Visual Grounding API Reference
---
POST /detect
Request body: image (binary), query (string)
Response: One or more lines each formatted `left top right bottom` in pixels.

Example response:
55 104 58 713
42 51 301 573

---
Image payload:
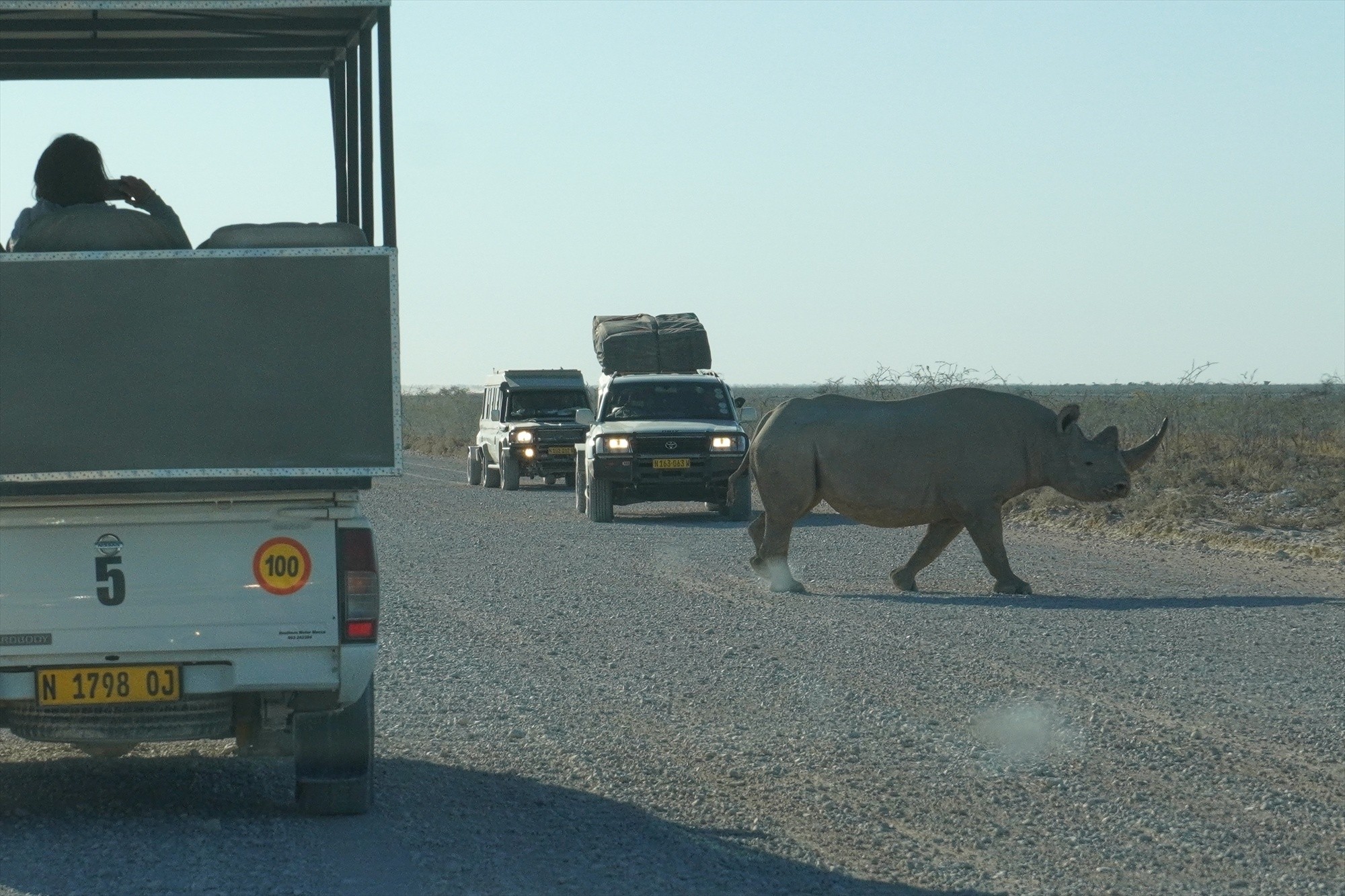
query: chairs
13 203 190 254
206 220 368 250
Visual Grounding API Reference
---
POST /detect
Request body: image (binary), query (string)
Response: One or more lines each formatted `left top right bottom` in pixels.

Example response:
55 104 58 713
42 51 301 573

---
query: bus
0 1 400 817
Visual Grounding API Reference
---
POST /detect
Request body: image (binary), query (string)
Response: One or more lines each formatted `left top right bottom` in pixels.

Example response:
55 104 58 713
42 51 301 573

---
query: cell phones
105 180 131 201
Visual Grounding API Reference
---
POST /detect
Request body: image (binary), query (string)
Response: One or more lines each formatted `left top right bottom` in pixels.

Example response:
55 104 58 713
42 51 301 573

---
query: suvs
573 369 753 523
467 368 595 491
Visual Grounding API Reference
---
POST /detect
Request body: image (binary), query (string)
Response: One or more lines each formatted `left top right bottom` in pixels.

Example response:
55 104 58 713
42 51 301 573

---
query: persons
5 133 192 252
612 388 656 417
